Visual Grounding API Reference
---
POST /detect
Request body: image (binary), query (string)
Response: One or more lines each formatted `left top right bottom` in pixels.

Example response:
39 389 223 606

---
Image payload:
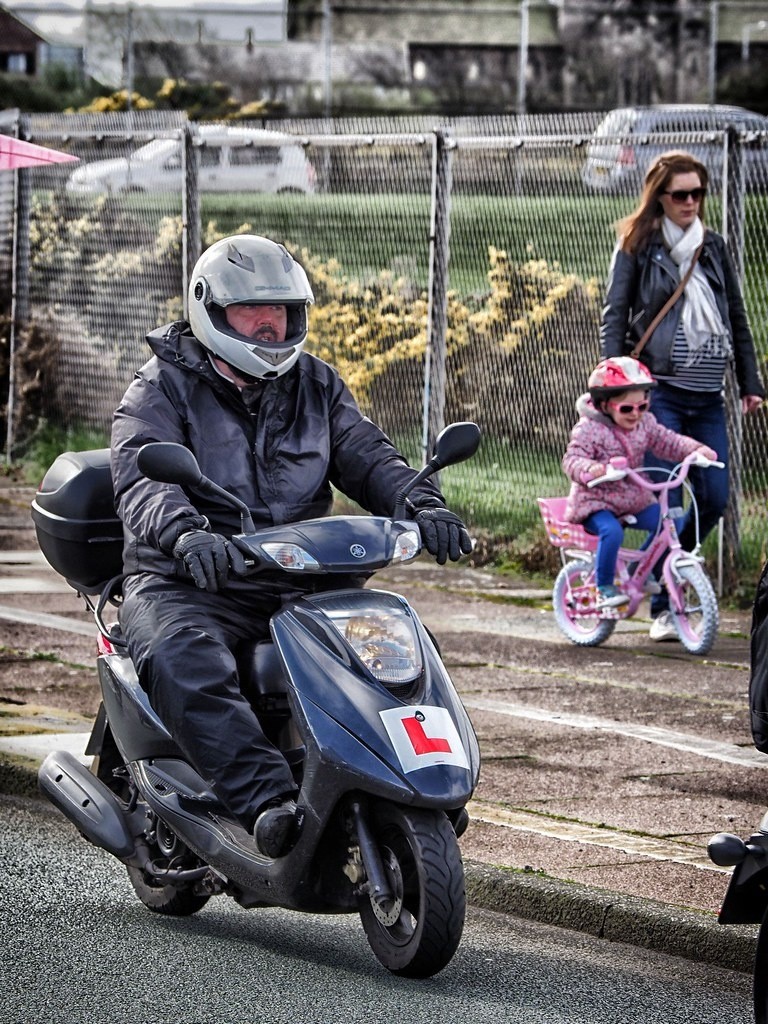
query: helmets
588 356 658 390
188 235 315 379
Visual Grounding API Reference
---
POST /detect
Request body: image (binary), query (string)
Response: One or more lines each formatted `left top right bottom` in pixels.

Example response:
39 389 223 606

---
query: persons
561 356 723 612
106 234 477 862
601 149 768 643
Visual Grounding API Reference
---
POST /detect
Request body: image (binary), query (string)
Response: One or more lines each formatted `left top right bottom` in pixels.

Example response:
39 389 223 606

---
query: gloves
172 528 247 591
413 508 472 566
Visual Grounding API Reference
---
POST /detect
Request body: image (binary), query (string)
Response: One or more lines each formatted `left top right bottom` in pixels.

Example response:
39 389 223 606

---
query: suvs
579 100 768 195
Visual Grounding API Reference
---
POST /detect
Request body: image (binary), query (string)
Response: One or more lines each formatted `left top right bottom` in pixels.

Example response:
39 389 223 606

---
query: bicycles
536 450 721 657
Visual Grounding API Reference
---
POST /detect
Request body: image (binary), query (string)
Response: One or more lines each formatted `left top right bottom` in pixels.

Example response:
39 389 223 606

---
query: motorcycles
32 422 483 979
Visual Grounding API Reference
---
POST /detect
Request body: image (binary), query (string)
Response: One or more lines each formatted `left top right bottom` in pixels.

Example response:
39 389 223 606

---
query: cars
65 125 316 215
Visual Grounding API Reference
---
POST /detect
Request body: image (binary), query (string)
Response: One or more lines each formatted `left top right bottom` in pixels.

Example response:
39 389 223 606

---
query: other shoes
452 807 469 838
254 795 302 859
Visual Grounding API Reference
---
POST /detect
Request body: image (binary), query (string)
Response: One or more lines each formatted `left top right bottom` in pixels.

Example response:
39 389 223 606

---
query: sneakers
627 562 661 593
650 609 681 641
595 584 630 611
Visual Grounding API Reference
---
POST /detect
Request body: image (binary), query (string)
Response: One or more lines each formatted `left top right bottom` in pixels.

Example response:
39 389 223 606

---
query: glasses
607 400 649 414
664 187 707 203
